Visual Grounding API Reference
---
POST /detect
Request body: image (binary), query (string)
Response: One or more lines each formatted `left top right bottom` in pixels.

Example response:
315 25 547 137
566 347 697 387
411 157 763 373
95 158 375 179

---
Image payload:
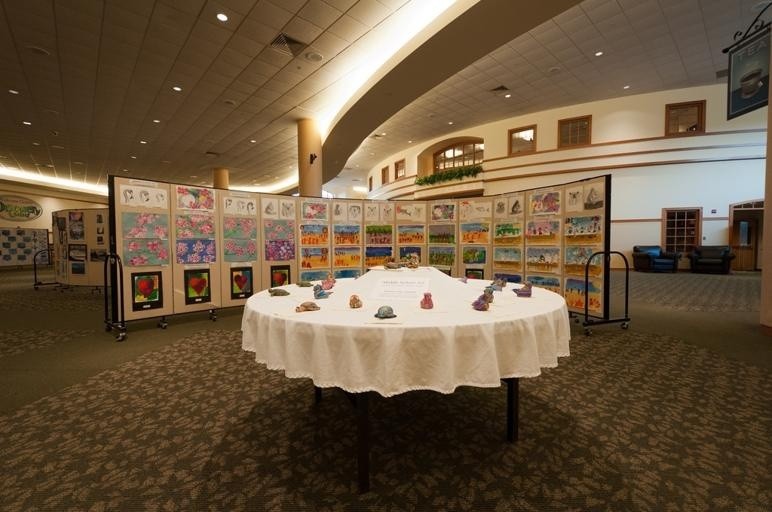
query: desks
240 266 572 443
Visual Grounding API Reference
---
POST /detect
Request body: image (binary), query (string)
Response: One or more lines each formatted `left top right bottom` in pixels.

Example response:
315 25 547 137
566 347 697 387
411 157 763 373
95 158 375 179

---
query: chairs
632 245 682 273
687 246 736 274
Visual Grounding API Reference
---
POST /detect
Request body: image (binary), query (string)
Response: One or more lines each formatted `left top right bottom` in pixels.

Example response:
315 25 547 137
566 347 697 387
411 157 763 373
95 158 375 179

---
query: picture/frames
726 27 771 123
